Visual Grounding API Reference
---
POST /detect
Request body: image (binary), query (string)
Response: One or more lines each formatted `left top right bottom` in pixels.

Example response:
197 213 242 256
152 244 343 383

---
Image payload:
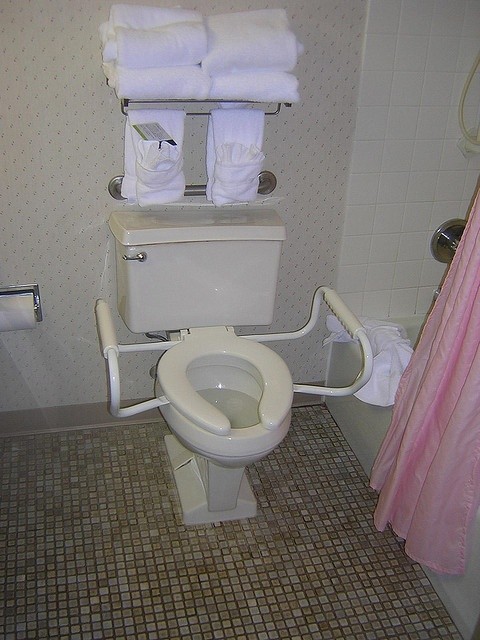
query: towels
199 62 302 109
96 3 206 40
100 58 209 102
321 309 424 408
201 5 299 45
204 107 268 208
119 107 189 208
198 33 303 73
96 22 206 75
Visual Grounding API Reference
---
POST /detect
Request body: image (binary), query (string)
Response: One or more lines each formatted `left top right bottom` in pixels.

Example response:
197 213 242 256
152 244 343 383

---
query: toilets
106 208 293 527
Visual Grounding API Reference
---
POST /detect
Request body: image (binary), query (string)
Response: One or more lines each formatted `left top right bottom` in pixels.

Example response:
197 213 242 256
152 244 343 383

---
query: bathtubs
324 313 480 638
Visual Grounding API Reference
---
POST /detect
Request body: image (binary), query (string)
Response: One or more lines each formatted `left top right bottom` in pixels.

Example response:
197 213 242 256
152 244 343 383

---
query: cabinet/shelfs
105 77 293 115
